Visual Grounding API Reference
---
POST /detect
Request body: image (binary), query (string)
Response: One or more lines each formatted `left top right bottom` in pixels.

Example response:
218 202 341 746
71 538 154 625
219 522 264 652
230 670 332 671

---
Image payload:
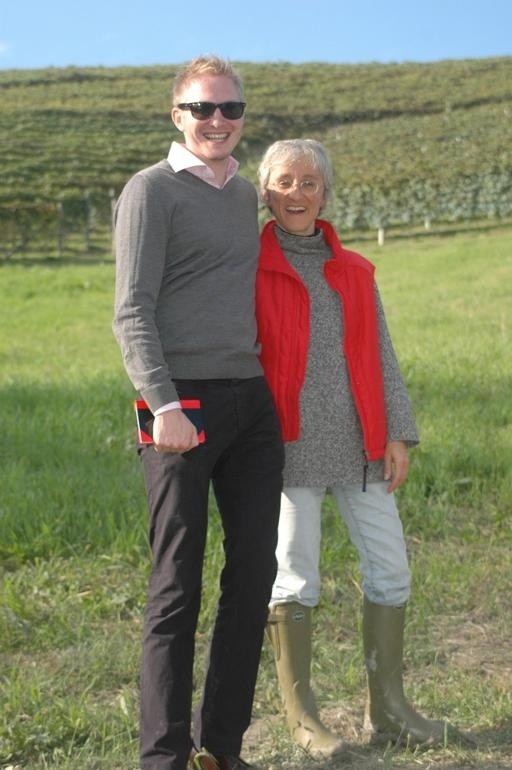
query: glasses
268 179 324 196
177 101 247 121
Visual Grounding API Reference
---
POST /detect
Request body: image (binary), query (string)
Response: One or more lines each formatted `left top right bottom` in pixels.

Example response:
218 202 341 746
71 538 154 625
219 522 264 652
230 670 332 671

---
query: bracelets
149 401 184 416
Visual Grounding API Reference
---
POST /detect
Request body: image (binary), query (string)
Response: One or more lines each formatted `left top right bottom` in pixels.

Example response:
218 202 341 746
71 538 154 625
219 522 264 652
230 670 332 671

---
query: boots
268 600 348 762
362 592 453 746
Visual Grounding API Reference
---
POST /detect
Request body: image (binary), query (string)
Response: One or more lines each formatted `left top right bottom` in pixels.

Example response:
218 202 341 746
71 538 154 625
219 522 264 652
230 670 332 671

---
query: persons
106 53 288 770
255 135 453 757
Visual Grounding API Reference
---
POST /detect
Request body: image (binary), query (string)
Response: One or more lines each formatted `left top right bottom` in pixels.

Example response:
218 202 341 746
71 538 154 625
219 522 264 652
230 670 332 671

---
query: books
133 399 206 446
